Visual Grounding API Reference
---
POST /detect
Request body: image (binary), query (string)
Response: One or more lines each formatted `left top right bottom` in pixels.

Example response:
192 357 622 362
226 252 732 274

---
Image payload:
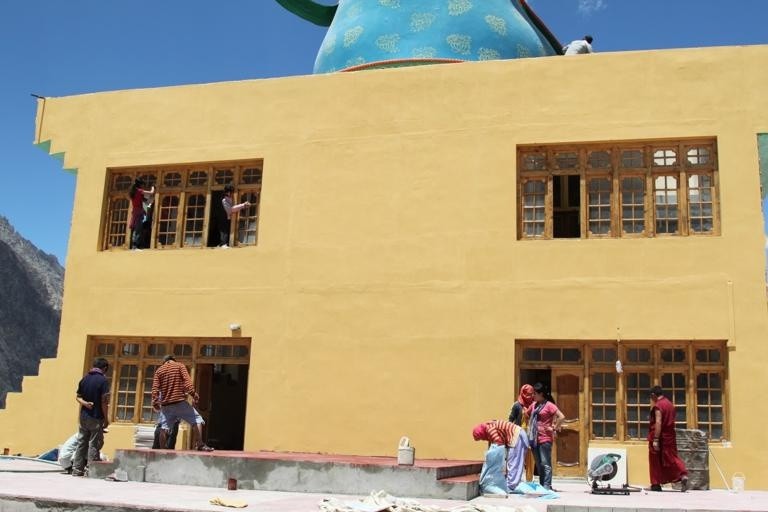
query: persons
129 177 155 250
71 358 110 476
508 384 536 482
58 429 109 474
473 420 530 491
152 354 214 452
525 382 565 489
562 35 593 54
643 386 689 492
220 184 251 246
152 411 178 448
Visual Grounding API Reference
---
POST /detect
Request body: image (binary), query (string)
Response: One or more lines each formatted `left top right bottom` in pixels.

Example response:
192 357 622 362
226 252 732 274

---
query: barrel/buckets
731 470 745 492
397 435 416 467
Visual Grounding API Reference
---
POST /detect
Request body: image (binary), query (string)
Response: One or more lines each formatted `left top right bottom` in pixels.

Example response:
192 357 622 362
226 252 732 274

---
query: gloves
153 404 161 412
192 393 200 406
652 439 660 452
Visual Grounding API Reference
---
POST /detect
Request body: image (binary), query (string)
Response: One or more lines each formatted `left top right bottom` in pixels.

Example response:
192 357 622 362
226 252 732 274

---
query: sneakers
71 471 84 476
643 485 662 491
196 443 214 451
681 474 689 492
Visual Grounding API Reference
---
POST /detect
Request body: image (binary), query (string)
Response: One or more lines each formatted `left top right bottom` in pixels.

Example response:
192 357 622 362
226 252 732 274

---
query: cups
4 448 9 455
228 479 237 490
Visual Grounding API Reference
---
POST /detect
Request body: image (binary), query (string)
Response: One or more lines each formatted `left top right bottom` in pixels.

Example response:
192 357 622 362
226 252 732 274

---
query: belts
163 400 185 406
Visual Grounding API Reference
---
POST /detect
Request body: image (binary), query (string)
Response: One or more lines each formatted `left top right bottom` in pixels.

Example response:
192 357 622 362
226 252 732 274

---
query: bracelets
552 426 555 431
653 437 659 439
654 439 660 442
